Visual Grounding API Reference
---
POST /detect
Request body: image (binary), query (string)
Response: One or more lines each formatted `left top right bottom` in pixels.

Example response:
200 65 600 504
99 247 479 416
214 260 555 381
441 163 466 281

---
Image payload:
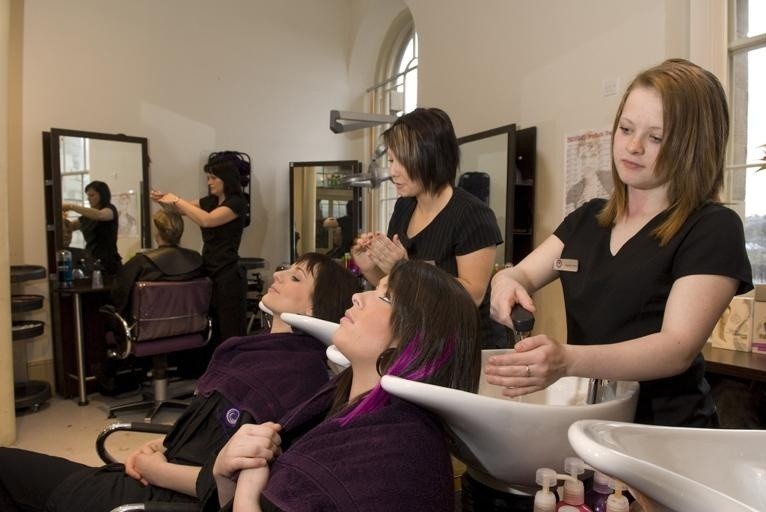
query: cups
56 249 73 288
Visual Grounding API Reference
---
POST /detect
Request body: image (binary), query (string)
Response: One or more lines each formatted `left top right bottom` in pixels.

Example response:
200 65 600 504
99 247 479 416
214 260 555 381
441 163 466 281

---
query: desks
47 273 104 408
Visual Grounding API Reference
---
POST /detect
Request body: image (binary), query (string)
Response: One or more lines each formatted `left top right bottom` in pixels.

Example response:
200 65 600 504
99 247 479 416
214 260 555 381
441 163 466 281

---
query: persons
19 254 355 512
61 220 85 269
150 155 259 349
566 135 618 217
492 56 753 427
349 107 503 309
59 181 124 272
322 197 355 256
107 204 203 341
210 258 479 508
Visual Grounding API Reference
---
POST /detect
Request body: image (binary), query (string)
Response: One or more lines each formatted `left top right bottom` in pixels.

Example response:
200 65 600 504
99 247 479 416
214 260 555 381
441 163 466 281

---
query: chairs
98 276 213 423
95 423 205 512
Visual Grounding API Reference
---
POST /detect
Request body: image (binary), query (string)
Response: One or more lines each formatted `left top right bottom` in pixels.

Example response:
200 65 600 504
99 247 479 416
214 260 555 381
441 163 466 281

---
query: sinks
326 345 639 485
568 420 766 512
259 302 338 347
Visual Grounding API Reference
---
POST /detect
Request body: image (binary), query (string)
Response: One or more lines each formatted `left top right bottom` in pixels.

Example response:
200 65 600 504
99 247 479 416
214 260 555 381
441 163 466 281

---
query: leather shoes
95 365 115 393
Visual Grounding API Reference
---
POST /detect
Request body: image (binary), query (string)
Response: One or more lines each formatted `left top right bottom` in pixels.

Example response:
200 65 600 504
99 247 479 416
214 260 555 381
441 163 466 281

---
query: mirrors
42 127 152 278
288 160 362 266
451 123 515 270
